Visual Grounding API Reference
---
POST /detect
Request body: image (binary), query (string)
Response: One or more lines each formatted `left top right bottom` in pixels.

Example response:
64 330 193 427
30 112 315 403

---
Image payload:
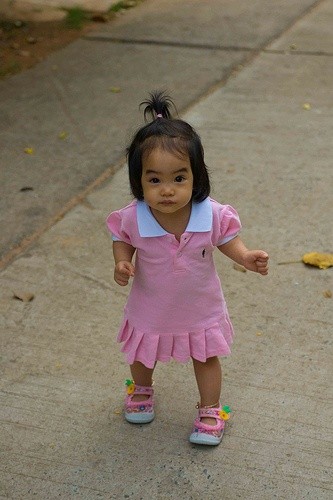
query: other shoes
123 380 155 424
189 402 232 445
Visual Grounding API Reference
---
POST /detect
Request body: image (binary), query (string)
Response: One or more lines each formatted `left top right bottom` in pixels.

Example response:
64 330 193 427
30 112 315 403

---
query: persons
104 87 271 445
